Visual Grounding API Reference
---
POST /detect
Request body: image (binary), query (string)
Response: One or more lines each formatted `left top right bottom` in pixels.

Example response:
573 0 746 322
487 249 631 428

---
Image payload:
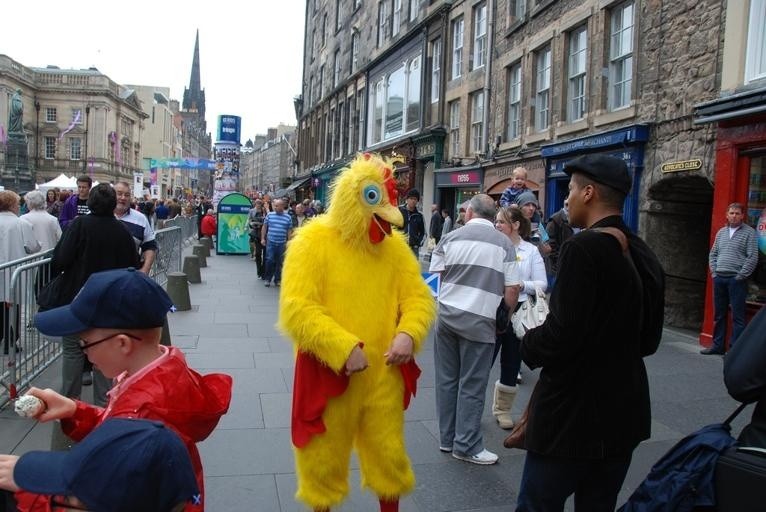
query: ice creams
15 395 47 417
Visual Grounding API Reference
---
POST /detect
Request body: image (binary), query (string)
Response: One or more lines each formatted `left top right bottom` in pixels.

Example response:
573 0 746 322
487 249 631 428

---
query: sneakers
440 446 499 464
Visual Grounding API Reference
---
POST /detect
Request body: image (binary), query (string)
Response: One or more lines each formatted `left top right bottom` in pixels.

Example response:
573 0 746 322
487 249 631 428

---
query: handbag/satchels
511 281 550 340
617 424 737 512
37 270 70 308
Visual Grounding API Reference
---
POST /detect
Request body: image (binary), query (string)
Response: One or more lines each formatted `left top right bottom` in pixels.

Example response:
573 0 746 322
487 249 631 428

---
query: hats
35 267 173 336
13 418 199 512
563 155 632 195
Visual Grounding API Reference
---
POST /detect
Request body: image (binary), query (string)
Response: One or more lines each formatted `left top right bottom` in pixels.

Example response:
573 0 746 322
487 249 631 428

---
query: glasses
78 333 141 354
50 495 87 512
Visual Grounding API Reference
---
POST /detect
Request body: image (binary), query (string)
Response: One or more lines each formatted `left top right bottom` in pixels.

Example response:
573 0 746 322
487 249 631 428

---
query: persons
1 268 234 511
51 181 160 456
129 193 196 230
430 204 467 259
700 204 759 355
500 167 581 294
247 188 323 288
279 157 435 511
196 192 217 247
6 86 26 133
491 206 548 428
723 305 766 512
430 194 521 467
399 188 427 260
0 176 93 354
521 155 667 512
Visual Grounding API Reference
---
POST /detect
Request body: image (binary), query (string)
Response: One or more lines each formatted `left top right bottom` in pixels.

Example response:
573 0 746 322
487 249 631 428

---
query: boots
492 380 518 429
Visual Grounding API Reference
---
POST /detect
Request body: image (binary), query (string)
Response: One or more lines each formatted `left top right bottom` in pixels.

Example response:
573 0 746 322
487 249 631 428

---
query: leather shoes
700 346 725 354
